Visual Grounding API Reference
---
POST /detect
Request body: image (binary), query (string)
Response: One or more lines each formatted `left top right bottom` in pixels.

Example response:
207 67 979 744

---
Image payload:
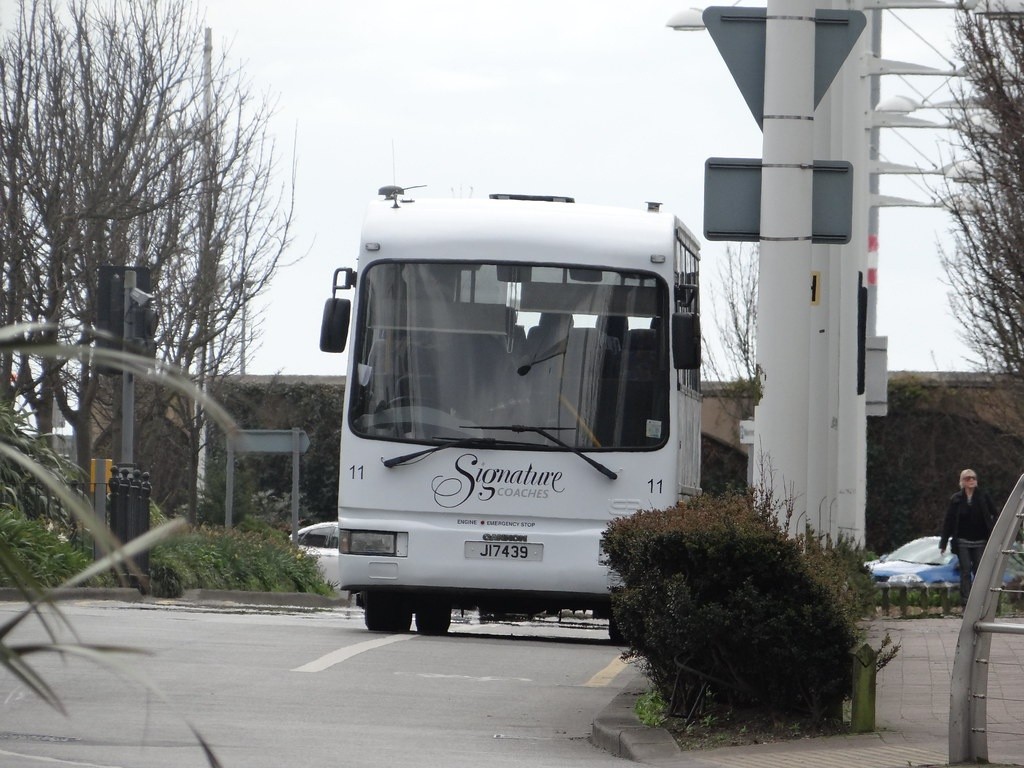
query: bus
319 179 701 651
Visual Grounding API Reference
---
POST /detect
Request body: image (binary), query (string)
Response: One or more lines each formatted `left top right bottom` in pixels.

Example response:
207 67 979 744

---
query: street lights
664 0 1023 568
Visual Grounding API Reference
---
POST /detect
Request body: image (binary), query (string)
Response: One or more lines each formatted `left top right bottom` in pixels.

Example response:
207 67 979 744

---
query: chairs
494 310 661 381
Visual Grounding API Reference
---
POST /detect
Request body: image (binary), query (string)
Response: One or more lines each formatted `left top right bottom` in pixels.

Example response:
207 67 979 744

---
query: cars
288 520 349 600
862 534 1021 590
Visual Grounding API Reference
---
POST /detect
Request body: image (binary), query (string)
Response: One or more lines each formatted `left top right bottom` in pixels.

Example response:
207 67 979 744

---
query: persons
940 469 999 620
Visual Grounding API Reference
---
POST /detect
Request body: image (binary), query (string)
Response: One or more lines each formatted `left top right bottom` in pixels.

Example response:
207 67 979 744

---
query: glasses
963 476 976 481
